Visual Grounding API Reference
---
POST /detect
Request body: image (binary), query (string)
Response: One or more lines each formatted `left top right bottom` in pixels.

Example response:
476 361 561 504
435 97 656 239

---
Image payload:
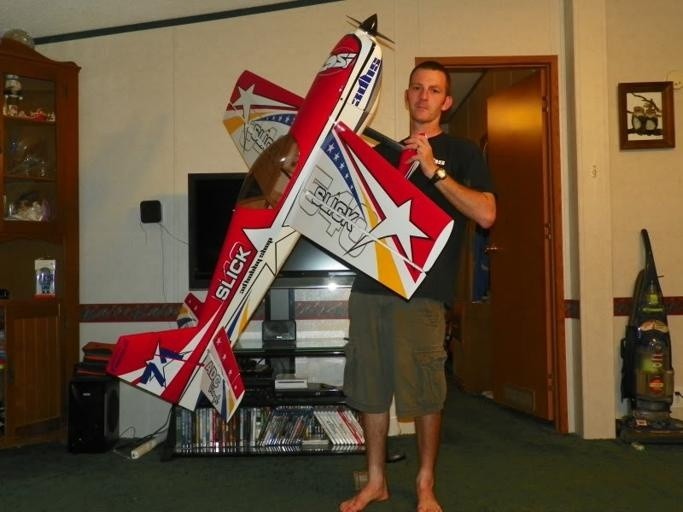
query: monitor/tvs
188 173 357 291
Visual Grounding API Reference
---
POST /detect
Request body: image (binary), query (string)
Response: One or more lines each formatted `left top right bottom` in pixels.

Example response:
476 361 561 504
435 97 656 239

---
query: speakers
67 375 119 454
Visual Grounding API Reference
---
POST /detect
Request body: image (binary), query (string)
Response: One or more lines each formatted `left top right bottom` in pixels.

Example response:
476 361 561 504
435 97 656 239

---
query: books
173 406 364 452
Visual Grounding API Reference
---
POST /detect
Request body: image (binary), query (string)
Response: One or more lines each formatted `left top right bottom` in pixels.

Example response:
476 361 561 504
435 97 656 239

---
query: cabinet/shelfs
0 36 79 450
169 338 368 461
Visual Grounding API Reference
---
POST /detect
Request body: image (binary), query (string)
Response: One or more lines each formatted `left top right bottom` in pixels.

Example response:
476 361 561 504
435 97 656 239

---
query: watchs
426 167 447 185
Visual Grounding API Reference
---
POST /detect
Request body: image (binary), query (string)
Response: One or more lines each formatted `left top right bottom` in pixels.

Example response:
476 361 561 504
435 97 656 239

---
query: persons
336 61 497 512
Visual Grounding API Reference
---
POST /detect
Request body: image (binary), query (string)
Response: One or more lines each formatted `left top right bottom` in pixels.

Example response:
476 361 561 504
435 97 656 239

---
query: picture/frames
617 80 676 150
